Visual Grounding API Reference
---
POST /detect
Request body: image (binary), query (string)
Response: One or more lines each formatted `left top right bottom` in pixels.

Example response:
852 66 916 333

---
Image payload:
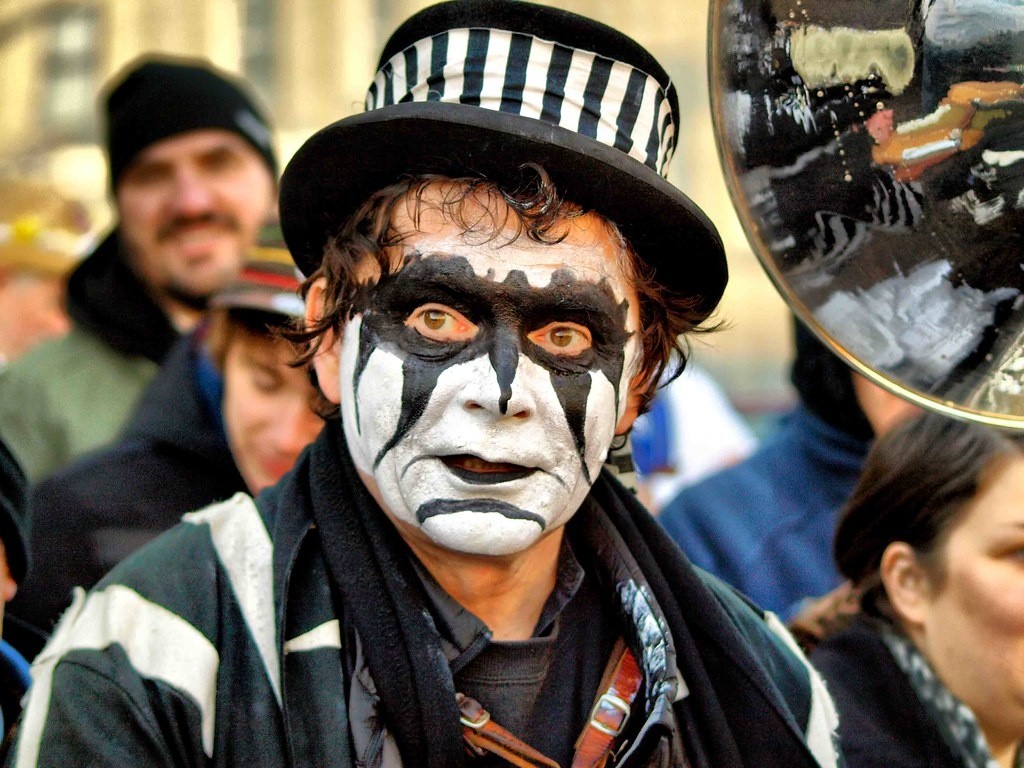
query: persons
0 1 919 768
647 306 918 628
782 403 1024 767
0 439 41 768
0 50 280 500
619 358 757 517
23 181 331 648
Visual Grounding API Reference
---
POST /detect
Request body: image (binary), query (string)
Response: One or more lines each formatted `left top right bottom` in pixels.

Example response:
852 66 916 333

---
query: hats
211 212 309 318
277 0 729 332
92 49 282 186
0 439 35 588
